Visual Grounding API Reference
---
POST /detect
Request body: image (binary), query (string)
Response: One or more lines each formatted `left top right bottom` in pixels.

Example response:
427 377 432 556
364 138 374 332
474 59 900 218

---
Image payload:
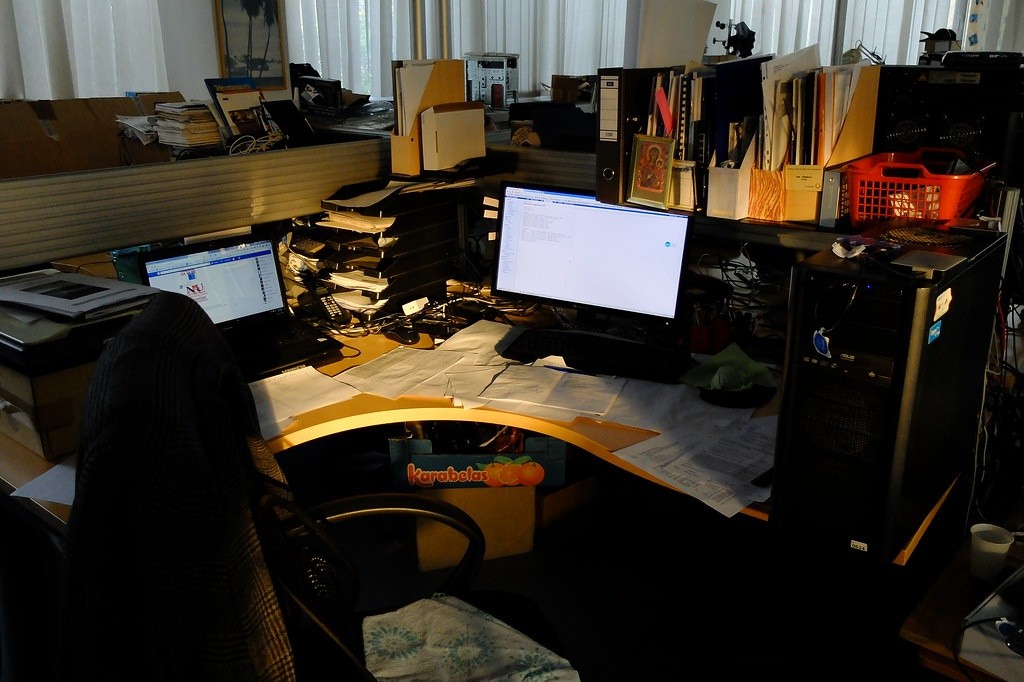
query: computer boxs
870 49 1024 194
467 55 519 111
768 217 1006 558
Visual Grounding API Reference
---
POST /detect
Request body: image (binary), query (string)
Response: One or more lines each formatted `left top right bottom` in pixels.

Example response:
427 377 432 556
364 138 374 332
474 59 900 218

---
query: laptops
138 233 344 384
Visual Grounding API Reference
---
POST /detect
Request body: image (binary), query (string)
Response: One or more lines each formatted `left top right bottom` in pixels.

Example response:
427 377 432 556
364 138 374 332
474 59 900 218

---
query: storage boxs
839 148 998 230
383 433 567 576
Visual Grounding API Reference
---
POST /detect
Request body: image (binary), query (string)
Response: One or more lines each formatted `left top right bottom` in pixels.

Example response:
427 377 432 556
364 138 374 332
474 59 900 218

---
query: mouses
385 326 420 345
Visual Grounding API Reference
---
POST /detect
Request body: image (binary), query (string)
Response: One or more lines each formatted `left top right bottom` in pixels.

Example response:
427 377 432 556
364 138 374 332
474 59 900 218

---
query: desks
309 116 393 137
0 281 964 566
899 538 1024 682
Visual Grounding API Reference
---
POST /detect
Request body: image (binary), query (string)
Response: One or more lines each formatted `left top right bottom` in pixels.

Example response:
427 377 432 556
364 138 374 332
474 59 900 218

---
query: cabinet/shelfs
325 181 489 323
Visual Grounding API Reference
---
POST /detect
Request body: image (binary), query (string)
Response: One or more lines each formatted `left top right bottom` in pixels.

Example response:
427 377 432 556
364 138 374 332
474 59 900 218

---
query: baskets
841 148 997 228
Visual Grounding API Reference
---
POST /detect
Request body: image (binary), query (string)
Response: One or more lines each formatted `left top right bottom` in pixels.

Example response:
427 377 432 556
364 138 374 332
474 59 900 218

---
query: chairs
67 291 580 682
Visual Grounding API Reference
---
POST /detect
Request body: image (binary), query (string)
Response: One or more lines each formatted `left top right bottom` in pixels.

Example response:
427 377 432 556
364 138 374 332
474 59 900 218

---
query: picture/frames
212 0 288 94
625 134 677 210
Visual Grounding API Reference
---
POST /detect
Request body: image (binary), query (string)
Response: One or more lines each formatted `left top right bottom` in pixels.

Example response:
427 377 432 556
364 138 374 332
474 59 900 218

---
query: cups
969 523 1015 583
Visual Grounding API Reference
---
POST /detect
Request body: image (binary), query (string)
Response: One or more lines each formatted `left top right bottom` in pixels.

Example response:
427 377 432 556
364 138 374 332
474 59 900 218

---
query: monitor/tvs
492 180 697 330
